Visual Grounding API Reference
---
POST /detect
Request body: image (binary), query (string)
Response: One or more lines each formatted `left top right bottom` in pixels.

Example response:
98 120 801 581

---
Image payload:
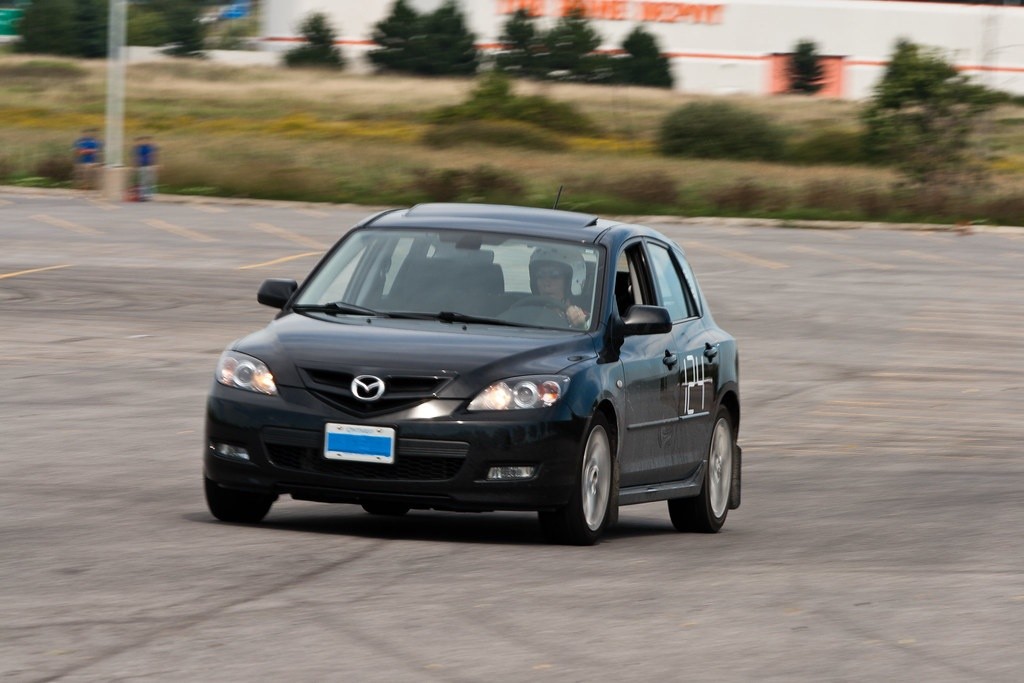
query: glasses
534 270 561 280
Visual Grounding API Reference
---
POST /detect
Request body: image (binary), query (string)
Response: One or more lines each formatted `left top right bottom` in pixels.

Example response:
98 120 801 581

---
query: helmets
529 244 586 297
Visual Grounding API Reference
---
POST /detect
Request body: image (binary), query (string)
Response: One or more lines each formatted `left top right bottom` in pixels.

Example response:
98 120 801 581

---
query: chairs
398 256 486 316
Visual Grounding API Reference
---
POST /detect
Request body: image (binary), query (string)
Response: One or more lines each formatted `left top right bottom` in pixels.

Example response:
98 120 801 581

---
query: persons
529 246 587 326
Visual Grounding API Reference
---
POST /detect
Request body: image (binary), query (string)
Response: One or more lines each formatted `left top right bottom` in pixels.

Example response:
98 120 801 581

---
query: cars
201 197 745 546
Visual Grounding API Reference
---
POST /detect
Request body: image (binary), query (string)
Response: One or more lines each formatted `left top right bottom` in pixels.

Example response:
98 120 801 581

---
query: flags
199 3 249 24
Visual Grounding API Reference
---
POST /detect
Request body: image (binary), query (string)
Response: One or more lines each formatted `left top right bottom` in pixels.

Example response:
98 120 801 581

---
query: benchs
470 263 633 321
